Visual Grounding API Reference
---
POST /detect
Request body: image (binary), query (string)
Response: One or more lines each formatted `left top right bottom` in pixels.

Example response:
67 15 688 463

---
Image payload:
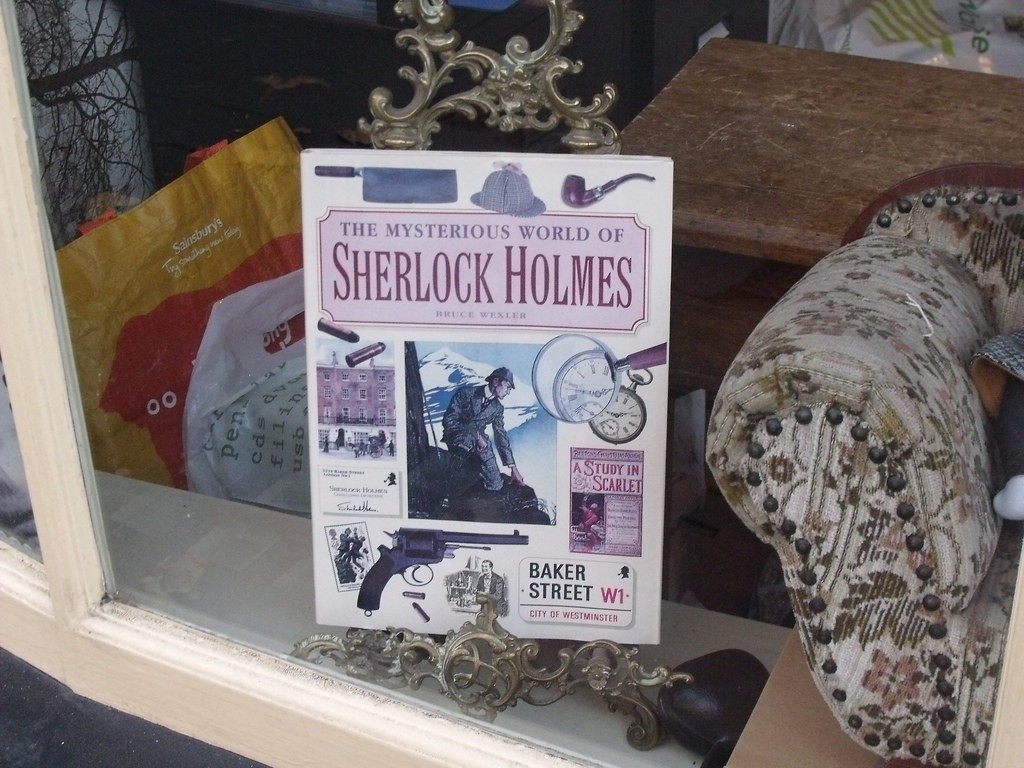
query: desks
618 34 1023 425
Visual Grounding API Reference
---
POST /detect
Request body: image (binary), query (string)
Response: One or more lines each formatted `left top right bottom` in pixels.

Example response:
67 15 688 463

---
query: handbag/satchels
778 0 1023 80
53 116 313 520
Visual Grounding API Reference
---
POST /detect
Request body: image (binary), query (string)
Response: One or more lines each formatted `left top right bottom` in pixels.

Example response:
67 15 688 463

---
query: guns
354 529 529 618
